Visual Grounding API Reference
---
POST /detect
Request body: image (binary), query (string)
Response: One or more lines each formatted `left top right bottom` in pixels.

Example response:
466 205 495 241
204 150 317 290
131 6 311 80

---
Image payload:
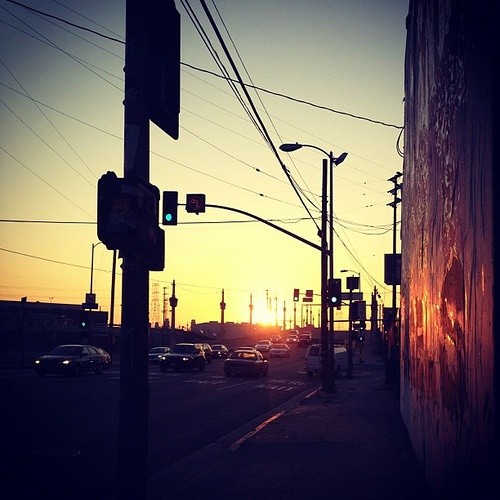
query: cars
269 334 283 343
269 343 290 357
148 346 170 365
34 344 106 376
224 347 268 379
286 329 314 348
255 340 273 352
210 344 228 358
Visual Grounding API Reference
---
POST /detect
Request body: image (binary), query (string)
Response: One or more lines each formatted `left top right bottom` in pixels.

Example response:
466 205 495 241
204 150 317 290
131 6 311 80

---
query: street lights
280 142 347 392
341 269 363 359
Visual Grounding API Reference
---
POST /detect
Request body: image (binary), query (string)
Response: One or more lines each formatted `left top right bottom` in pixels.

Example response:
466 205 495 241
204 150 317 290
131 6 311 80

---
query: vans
304 344 348 377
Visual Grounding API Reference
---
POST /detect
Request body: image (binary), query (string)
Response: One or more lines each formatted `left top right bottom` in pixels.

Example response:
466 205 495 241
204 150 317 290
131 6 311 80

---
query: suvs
159 343 205 372
193 342 213 363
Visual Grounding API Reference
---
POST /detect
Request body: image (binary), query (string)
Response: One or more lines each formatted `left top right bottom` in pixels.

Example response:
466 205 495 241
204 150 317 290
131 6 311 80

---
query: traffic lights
163 191 178 225
327 279 341 307
187 194 205 213
